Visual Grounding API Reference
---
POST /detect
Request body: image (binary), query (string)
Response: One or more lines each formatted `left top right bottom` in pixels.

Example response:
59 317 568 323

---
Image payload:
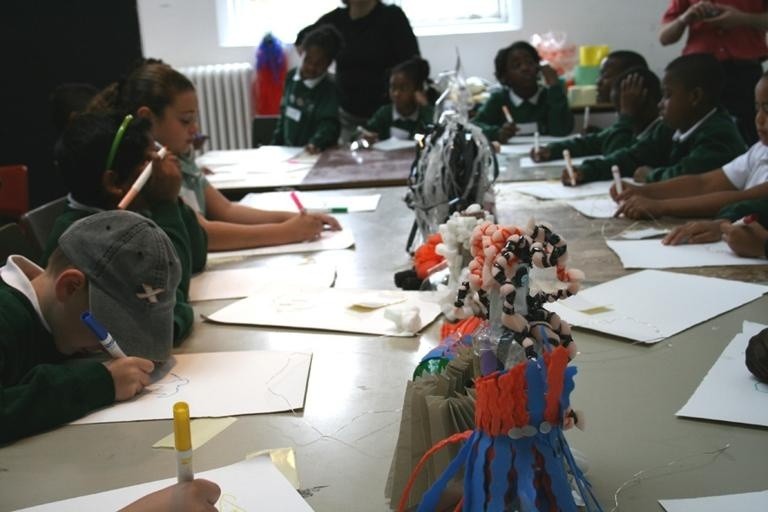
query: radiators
178 63 254 155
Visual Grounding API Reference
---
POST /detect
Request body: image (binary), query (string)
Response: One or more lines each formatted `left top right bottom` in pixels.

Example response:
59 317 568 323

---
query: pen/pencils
501 105 514 123
290 192 347 214
356 126 372 136
731 215 755 226
582 106 590 132
289 160 314 164
563 149 576 187
612 165 624 206
173 401 193 483
534 131 541 163
81 312 125 360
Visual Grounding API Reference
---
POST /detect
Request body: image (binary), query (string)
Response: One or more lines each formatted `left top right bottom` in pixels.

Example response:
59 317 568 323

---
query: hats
59 210 182 362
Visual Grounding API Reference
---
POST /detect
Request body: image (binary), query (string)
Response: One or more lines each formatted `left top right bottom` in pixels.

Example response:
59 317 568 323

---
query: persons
349 57 436 154
593 49 649 110
658 0 768 63
265 23 347 153
611 75 767 220
1 210 195 450
662 196 766 262
45 108 209 351
113 478 222 512
292 1 423 148
744 328 767 387
529 66 663 171
466 41 570 143
75 56 342 251
562 52 747 185
